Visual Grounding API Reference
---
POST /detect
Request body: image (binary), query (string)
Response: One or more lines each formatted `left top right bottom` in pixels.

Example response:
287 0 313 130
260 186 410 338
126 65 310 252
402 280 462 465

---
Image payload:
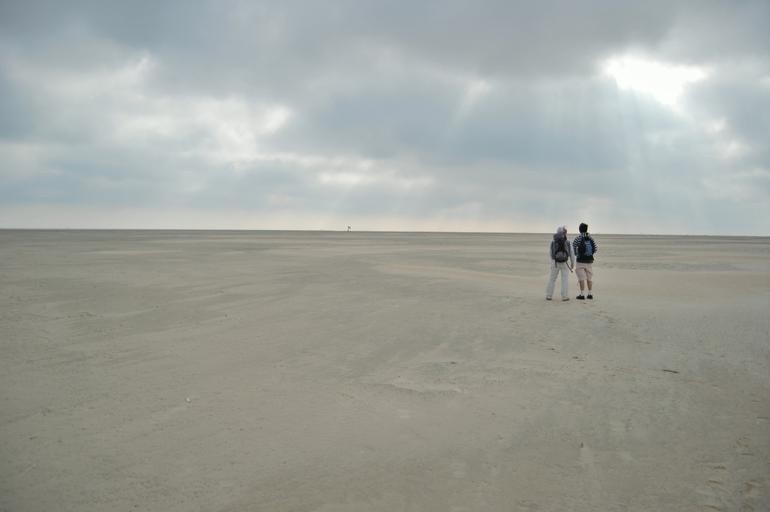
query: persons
545 222 598 301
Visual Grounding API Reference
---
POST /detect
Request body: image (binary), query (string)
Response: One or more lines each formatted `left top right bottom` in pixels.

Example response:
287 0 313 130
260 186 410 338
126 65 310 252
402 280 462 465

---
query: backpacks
555 239 568 262
578 237 595 260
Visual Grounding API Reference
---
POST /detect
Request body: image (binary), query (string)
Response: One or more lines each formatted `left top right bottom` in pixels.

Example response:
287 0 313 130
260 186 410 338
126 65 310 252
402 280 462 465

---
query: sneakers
576 294 593 299
546 296 569 301
